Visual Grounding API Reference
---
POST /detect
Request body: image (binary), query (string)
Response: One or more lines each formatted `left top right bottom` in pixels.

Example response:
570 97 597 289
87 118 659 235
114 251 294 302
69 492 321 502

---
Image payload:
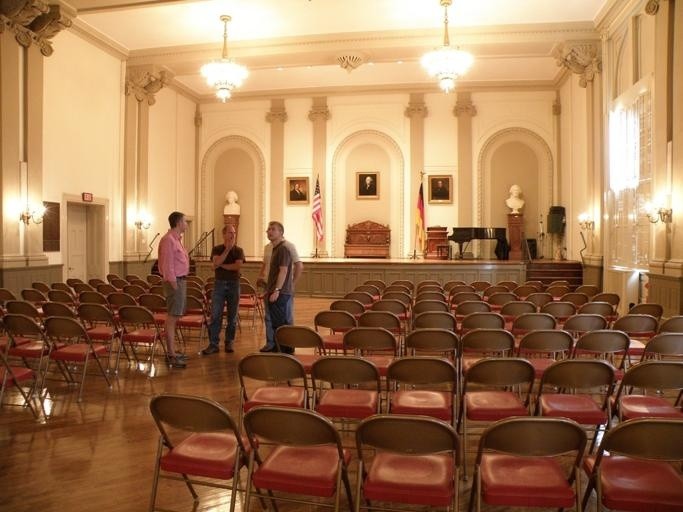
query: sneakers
203 344 219 355
260 345 277 352
226 342 233 353
165 353 189 368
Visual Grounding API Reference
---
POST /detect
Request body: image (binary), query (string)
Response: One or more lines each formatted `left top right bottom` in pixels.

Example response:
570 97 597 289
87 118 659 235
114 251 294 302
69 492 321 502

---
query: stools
437 245 452 259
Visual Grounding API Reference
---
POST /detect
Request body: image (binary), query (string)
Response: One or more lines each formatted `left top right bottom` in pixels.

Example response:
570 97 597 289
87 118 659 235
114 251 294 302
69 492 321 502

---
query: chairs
0 272 261 425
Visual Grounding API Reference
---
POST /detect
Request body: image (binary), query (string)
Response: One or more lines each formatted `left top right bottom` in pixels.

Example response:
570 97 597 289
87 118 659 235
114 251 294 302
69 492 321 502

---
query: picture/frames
427 174 453 205
286 176 310 206
356 172 380 199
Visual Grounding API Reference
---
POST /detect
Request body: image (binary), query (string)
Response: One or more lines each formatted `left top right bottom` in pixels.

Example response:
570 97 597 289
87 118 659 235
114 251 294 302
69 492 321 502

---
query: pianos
448 226 509 260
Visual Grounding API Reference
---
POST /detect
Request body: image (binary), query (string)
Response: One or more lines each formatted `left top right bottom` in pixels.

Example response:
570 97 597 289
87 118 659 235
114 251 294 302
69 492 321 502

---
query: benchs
344 220 391 259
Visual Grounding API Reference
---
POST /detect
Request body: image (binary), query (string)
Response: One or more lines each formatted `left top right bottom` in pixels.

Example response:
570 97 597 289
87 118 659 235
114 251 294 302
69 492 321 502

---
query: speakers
547 206 565 233
524 238 537 260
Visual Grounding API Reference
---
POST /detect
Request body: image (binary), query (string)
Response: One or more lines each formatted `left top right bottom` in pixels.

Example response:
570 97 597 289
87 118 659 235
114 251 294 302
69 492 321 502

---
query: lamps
200 14 251 104
419 0 477 93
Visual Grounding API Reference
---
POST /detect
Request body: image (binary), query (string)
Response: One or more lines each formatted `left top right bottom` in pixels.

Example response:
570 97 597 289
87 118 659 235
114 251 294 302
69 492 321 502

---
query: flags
311 173 324 240
415 179 427 252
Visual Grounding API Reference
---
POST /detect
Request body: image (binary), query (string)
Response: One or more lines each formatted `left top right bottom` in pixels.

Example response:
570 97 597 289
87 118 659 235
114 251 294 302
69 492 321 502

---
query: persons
256 221 304 354
201 223 246 355
291 183 305 200
433 180 448 199
222 191 240 215
266 222 294 353
504 185 525 209
363 176 375 195
156 212 190 368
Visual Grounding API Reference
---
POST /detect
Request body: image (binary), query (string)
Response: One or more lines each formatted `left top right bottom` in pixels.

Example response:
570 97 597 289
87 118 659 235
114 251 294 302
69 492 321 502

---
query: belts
176 276 186 281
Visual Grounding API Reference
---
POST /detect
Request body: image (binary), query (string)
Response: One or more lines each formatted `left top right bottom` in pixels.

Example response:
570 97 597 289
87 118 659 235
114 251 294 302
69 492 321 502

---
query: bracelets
274 288 281 291
